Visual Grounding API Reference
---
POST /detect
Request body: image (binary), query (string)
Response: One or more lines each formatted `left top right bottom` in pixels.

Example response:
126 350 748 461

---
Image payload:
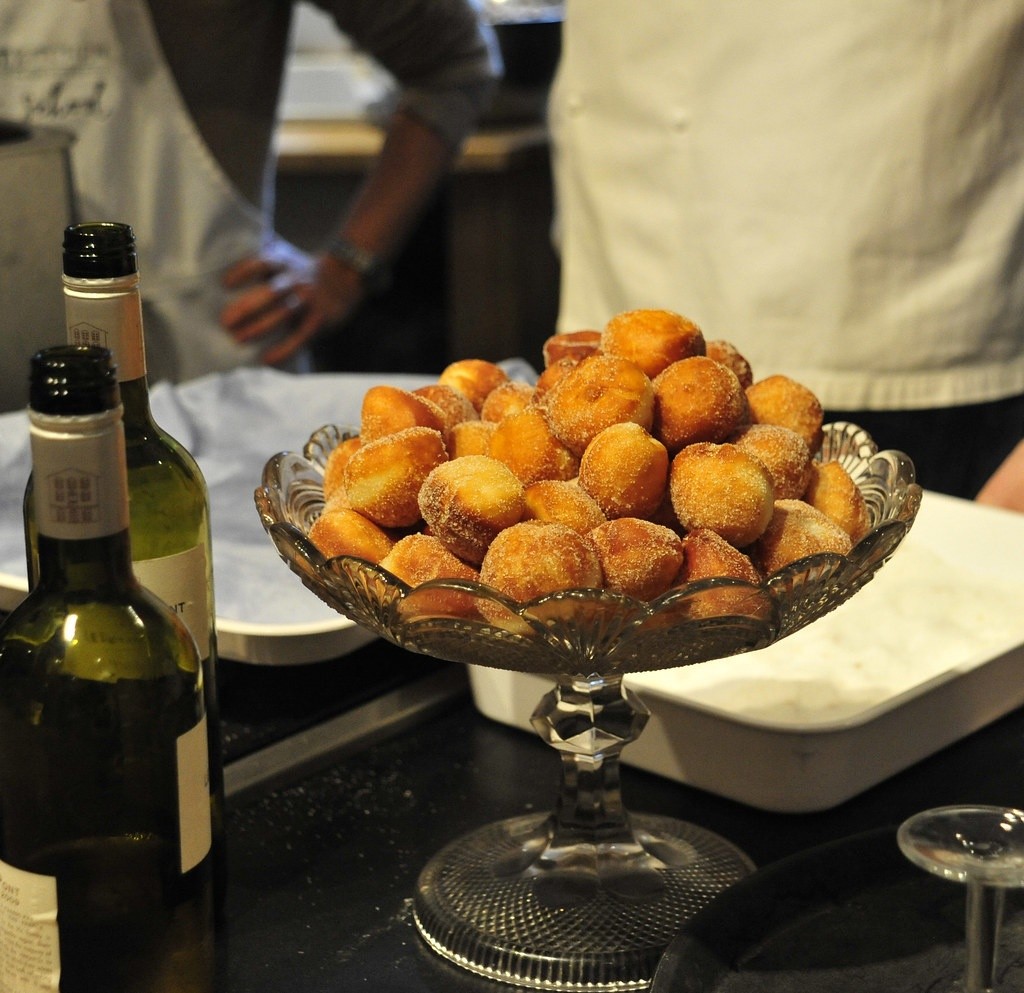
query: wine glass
895 804 1024 993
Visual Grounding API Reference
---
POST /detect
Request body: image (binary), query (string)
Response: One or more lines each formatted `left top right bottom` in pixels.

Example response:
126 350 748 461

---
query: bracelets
321 234 389 286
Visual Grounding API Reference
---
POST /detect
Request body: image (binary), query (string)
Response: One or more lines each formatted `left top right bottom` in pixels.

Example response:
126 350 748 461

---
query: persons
0 0 505 414
544 0 1023 521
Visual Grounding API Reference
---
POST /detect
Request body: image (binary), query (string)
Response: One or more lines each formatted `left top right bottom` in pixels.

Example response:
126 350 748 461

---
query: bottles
20 218 225 820
0 342 220 993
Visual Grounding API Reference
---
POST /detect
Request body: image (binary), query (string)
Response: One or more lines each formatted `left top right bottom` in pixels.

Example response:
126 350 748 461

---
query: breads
310 309 867 643
